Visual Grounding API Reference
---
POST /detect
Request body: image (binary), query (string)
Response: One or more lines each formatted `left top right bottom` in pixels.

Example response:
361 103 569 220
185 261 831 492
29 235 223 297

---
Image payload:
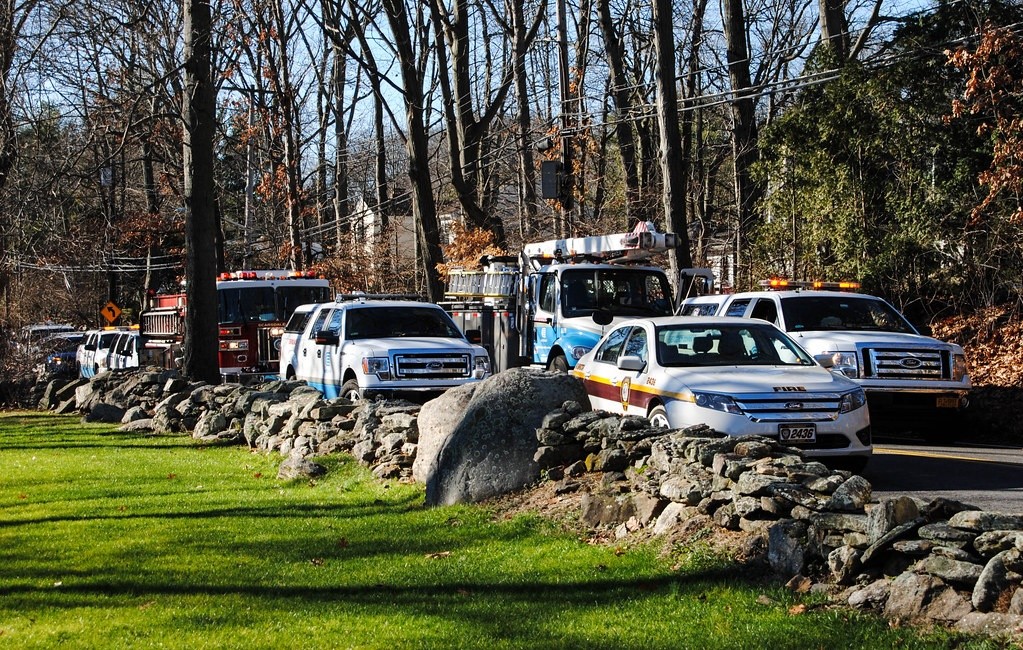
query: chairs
568 281 591 308
691 337 721 360
659 341 689 362
718 332 751 361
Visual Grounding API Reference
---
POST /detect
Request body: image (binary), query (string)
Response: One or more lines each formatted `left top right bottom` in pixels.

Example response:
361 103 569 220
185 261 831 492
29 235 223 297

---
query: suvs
16 323 142 380
662 278 973 432
277 291 494 405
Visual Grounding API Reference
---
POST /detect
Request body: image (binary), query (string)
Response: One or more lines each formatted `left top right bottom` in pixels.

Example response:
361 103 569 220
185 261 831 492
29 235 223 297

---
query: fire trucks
570 315 873 481
437 219 715 375
135 269 336 386
